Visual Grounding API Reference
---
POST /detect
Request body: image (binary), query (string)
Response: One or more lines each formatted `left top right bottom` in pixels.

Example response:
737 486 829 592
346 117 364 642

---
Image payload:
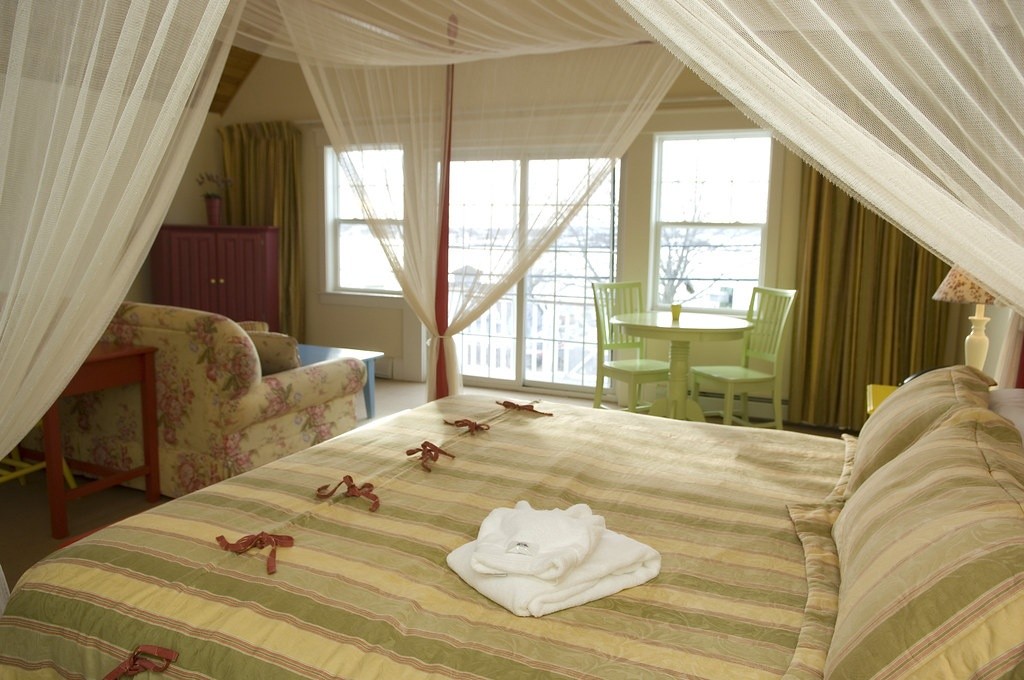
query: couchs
21 299 368 499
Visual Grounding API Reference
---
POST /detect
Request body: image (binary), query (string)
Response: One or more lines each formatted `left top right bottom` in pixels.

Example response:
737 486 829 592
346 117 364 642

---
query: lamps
931 262 1012 372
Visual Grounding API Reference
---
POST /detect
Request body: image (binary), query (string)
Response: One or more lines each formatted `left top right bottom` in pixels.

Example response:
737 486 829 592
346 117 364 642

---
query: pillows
786 406 1024 680
822 364 998 499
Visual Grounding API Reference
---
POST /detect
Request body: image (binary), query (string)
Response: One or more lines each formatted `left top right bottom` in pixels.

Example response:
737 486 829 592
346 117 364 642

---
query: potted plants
196 172 234 226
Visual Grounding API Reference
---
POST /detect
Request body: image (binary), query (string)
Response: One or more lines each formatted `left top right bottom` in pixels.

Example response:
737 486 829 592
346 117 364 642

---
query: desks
608 311 756 422
297 342 386 419
44 342 163 537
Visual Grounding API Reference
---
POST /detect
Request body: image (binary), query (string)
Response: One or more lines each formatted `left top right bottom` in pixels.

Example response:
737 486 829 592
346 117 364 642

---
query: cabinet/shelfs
152 225 281 332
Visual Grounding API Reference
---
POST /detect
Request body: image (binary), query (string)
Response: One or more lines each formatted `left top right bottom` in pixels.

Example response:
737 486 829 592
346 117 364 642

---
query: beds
0 395 847 680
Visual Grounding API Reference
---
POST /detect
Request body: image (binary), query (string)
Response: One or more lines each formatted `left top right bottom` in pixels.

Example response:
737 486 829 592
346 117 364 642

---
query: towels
445 500 662 618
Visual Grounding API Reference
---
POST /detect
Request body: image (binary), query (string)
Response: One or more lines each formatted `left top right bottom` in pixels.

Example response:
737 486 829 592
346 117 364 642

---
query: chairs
590 281 670 414
690 286 799 430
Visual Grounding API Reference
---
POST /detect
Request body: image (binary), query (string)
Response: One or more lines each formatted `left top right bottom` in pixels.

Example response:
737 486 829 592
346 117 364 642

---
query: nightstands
865 384 899 414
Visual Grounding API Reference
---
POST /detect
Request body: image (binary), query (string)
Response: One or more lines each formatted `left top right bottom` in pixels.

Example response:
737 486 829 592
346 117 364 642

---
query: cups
671 304 681 320
